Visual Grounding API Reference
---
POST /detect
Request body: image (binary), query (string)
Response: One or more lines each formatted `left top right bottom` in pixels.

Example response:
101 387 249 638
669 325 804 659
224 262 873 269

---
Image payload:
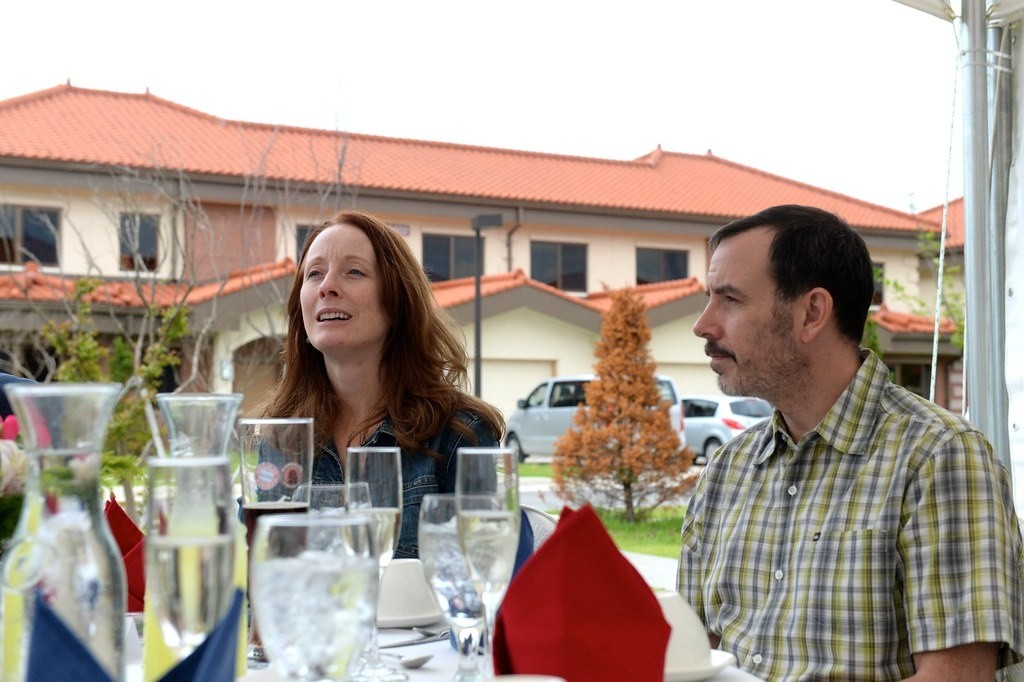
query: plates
662 649 737 682
376 613 443 627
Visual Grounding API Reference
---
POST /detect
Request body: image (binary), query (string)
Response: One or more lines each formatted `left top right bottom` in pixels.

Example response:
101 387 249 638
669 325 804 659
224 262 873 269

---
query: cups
378 559 434 617
144 417 401 682
652 588 710 668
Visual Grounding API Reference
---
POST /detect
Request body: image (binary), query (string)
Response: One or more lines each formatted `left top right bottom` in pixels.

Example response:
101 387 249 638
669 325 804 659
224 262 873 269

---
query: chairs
692 405 705 416
554 388 574 406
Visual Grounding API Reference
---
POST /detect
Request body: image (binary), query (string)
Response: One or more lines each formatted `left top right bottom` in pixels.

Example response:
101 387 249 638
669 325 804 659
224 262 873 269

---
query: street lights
473 214 502 400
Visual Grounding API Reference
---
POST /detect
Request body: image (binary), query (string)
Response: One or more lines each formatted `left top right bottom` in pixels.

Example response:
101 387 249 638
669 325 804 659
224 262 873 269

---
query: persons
677 204 1024 682
256 211 534 580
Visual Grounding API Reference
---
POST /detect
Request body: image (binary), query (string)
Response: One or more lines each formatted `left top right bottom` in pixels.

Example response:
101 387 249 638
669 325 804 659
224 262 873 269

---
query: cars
682 396 775 464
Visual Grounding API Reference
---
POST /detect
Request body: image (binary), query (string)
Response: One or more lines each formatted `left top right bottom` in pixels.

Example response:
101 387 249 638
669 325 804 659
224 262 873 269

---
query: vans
504 378 686 463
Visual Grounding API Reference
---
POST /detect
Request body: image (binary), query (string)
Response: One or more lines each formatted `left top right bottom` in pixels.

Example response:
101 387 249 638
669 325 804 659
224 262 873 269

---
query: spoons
378 652 433 668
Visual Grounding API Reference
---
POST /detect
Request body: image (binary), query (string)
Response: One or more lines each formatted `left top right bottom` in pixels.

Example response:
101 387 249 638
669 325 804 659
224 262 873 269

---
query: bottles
156 392 243 539
0 384 127 682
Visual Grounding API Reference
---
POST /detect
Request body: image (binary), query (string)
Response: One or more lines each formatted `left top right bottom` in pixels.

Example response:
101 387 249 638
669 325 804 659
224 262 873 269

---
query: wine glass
418 494 496 682
457 448 521 680
